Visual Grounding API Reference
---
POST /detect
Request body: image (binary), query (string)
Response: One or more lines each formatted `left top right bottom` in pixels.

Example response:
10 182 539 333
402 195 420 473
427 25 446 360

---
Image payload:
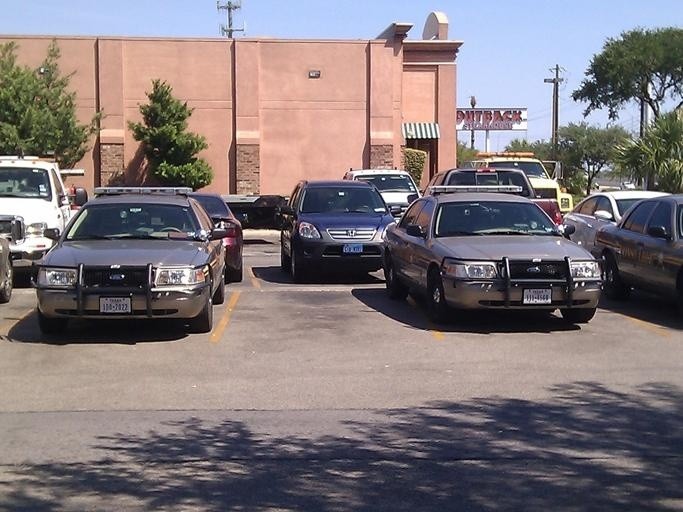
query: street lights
543 78 565 144
469 96 477 151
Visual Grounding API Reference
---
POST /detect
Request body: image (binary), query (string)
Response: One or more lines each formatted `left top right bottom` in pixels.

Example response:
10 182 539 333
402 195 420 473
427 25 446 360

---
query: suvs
343 166 424 221
468 152 564 215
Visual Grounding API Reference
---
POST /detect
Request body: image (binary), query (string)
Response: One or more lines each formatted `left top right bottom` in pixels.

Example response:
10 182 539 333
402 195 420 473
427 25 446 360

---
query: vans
280 179 399 278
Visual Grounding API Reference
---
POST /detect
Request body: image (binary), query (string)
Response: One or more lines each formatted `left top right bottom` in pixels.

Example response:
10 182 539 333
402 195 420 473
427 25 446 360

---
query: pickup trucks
406 167 565 227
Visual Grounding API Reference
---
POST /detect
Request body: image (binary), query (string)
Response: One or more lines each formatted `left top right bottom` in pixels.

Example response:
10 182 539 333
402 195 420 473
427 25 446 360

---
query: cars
561 190 673 255
589 193 683 310
379 193 603 320
